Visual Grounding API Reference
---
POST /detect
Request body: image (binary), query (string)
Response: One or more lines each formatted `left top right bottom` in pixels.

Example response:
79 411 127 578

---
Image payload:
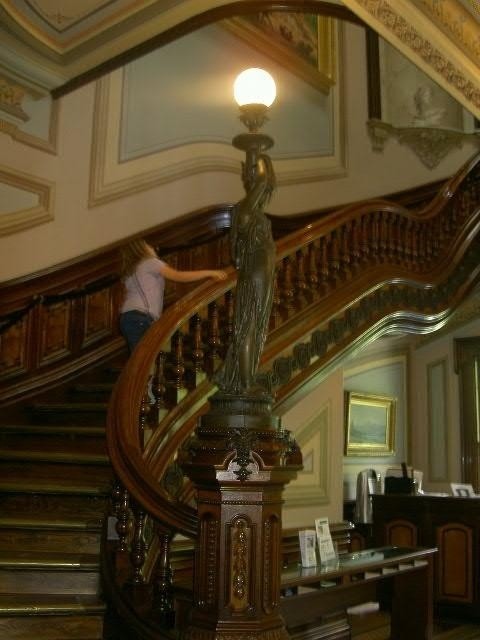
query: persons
115 237 228 405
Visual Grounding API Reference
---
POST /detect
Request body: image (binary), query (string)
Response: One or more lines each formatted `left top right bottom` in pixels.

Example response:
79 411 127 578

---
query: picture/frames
222 12 340 97
344 391 399 458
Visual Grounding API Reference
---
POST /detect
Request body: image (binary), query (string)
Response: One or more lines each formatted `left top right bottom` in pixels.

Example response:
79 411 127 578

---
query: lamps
199 66 281 427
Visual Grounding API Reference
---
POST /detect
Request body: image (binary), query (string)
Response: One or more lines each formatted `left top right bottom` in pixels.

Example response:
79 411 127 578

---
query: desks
279 546 439 639
